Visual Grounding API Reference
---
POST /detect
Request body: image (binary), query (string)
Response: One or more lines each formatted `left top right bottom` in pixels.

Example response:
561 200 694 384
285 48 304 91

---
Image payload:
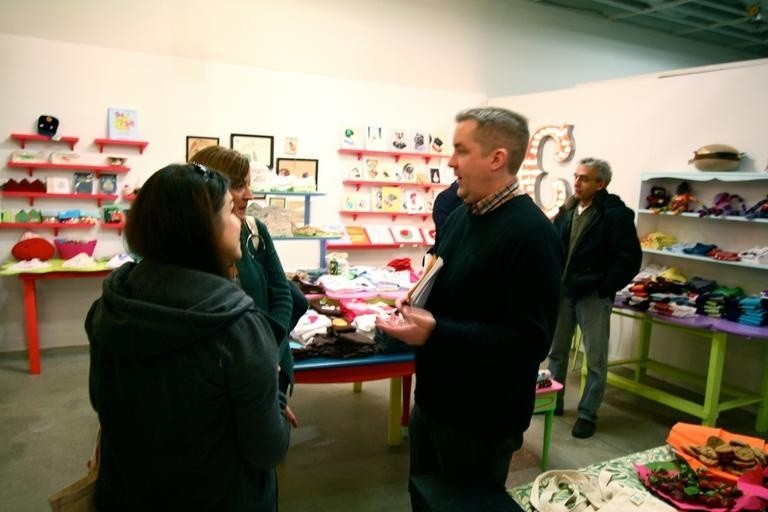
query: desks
299 275 418 394
501 438 713 512
0 251 117 376
532 373 564 479
577 300 767 433
291 352 416 449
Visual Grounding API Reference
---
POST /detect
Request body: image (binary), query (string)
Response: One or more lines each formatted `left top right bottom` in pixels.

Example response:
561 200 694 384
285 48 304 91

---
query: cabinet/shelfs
625 168 768 272
244 190 343 270
0 128 151 239
323 140 454 256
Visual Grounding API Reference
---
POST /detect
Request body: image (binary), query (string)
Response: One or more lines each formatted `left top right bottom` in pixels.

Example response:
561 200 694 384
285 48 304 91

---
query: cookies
681 435 768 476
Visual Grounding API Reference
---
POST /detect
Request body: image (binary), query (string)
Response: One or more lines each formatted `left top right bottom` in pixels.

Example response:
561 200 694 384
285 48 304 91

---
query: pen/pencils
351 271 367 280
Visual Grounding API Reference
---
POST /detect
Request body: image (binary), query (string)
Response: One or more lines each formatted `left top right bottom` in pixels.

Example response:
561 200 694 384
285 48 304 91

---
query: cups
99 173 117 194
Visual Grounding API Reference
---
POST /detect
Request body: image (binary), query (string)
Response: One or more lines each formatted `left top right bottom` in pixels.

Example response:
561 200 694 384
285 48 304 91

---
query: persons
84 162 298 512
547 157 643 438
188 145 296 397
375 106 564 511
432 180 464 266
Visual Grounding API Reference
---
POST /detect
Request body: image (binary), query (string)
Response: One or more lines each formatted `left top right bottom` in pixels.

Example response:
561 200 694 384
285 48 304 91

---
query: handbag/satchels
268 281 308 335
47 429 101 512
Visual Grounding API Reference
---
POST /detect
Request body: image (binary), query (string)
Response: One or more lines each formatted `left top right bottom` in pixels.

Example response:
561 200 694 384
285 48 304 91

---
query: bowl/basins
12 238 54 261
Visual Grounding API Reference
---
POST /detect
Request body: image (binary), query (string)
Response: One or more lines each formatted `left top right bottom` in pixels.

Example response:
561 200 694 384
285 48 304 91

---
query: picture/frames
106 107 139 141
274 156 320 192
228 132 276 170
185 135 220 165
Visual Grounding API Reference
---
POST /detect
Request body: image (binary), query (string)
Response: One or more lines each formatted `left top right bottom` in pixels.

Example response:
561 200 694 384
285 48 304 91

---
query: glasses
192 161 210 183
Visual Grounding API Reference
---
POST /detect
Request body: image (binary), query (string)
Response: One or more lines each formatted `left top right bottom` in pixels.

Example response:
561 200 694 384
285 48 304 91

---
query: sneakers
572 418 595 438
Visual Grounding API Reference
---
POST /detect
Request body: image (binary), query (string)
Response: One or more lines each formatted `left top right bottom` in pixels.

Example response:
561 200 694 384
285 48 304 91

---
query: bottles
338 256 349 275
71 172 93 192
329 257 338 275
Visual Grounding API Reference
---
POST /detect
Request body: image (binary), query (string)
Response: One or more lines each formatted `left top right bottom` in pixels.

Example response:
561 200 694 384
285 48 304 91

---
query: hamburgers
689 145 747 172
